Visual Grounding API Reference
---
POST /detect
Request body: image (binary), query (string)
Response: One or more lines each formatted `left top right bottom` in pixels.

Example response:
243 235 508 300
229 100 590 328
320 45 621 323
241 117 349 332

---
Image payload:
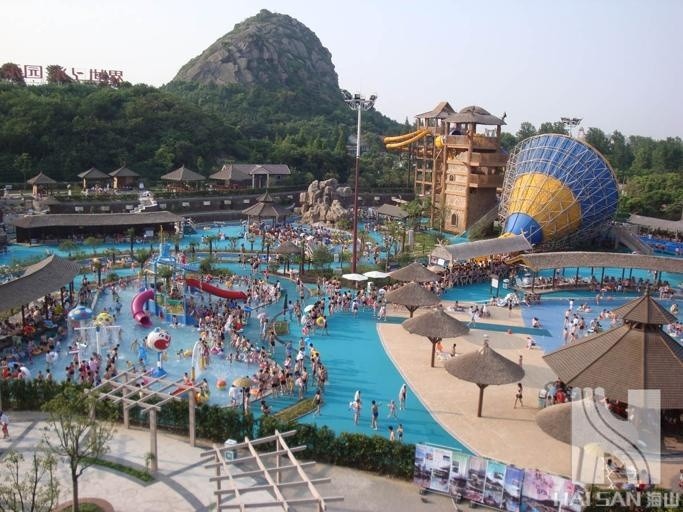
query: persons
1 178 683 448
